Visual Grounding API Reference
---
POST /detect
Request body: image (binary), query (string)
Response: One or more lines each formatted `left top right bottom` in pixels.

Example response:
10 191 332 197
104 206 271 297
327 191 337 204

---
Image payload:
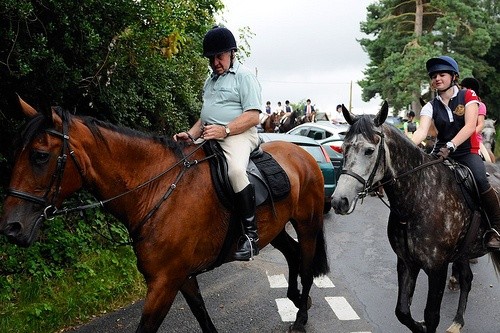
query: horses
261 108 316 133
331 99 499 333
1 91 329 333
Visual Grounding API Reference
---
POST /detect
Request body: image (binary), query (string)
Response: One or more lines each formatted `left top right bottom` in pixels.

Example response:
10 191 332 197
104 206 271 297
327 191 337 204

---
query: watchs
223 124 231 137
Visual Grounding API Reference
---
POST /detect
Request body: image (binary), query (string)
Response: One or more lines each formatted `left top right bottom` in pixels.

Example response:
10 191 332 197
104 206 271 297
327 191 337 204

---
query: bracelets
185 131 196 141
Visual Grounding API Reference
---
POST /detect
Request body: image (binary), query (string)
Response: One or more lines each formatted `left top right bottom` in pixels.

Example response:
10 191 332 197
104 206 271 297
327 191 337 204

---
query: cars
259 133 337 214
284 121 347 180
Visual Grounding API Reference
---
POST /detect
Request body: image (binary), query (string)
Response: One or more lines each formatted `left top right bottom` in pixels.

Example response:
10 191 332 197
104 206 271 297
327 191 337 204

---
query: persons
173 27 262 260
285 99 293 113
265 100 272 117
331 104 347 123
304 98 315 116
276 101 284 115
404 56 500 248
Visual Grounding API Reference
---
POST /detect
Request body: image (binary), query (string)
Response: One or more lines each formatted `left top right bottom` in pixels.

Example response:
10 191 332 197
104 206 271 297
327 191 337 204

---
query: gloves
437 146 451 160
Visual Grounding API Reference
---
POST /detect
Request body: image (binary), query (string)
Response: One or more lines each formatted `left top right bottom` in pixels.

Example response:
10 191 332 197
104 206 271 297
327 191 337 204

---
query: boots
233 184 259 258
481 187 500 247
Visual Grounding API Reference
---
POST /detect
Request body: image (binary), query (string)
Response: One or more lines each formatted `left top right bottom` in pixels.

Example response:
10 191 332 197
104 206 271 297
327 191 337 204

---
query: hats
461 77 479 93
203 26 237 57
426 55 458 80
406 110 415 116
